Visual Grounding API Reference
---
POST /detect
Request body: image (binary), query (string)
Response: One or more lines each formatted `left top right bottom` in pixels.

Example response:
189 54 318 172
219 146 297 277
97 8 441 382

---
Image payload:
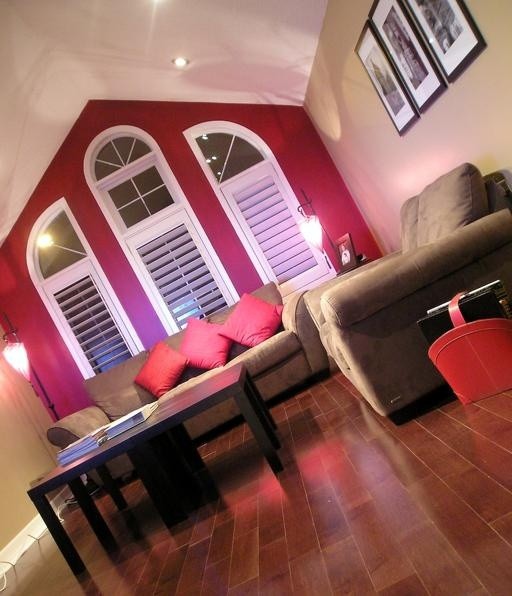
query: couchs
46 289 330 493
302 169 511 426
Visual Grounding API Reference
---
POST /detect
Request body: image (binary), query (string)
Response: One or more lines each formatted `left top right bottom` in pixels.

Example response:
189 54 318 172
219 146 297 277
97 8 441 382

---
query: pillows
399 191 420 255
81 281 286 422
416 163 490 247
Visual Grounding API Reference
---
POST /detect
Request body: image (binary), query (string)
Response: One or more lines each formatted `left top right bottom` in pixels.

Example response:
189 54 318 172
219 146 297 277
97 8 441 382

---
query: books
56 400 160 468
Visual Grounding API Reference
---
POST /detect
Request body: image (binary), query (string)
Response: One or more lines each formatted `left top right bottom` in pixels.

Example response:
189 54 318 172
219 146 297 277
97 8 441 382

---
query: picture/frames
404 0 487 84
355 19 420 137
368 0 448 114
333 233 360 274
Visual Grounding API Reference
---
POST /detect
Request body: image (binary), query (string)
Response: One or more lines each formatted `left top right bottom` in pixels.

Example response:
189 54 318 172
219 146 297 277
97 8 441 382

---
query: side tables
336 258 371 276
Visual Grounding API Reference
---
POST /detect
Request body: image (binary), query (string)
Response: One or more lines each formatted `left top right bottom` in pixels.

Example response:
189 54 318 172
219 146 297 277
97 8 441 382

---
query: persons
343 253 349 265
340 244 351 268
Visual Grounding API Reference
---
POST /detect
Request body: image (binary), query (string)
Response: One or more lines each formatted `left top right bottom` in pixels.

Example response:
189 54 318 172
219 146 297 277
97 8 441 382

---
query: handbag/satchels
427 293 511 406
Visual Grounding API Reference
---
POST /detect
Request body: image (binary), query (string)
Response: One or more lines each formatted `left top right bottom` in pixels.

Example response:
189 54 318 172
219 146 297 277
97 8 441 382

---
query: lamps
0 313 99 505
296 188 334 266
